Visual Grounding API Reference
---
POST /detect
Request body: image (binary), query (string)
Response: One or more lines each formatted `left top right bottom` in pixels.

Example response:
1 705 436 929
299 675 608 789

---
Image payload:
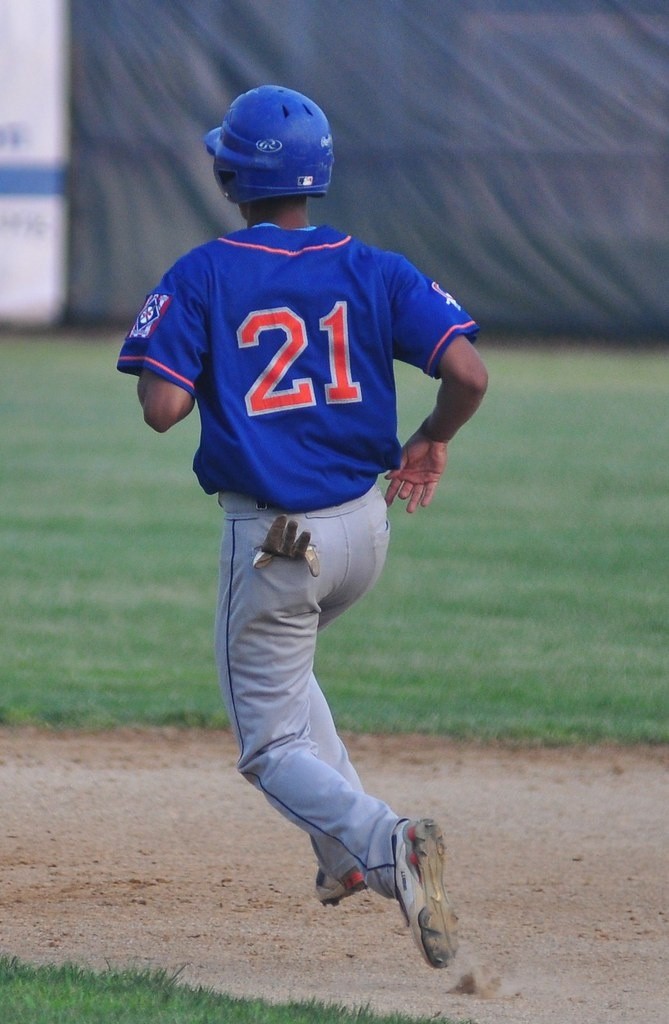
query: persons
117 84 488 966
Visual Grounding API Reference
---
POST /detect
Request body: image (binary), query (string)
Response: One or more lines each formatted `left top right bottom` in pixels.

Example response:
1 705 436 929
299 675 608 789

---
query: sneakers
390 815 459 969
315 864 367 907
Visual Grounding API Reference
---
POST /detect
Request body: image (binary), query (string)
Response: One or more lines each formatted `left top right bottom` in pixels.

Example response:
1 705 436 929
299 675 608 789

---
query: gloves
252 514 321 578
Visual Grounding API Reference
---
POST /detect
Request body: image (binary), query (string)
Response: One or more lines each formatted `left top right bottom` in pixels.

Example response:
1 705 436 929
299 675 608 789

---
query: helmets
204 85 334 203
